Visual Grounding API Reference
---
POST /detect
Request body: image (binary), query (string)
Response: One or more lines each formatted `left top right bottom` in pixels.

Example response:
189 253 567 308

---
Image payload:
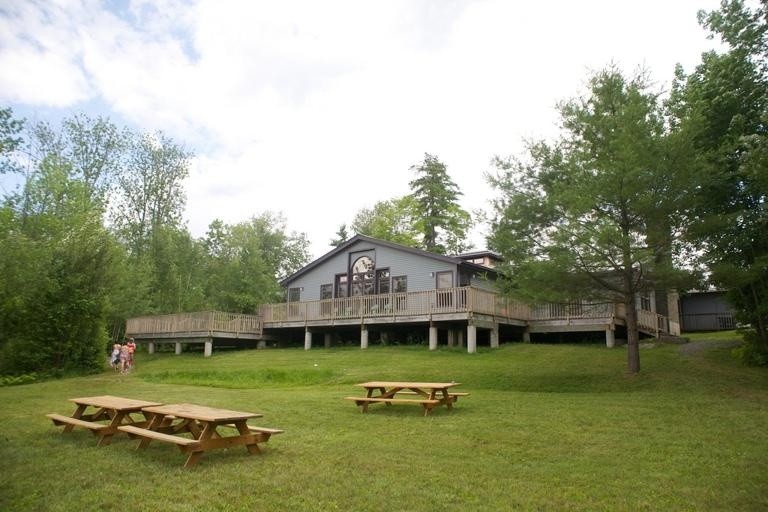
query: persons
110 337 136 373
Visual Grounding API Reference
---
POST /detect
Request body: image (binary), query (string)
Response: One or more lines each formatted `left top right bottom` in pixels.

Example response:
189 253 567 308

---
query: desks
61 395 263 468
354 381 462 416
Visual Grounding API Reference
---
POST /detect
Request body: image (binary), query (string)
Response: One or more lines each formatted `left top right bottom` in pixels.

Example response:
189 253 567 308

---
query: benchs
45 413 114 447
223 424 284 455
344 392 471 417
